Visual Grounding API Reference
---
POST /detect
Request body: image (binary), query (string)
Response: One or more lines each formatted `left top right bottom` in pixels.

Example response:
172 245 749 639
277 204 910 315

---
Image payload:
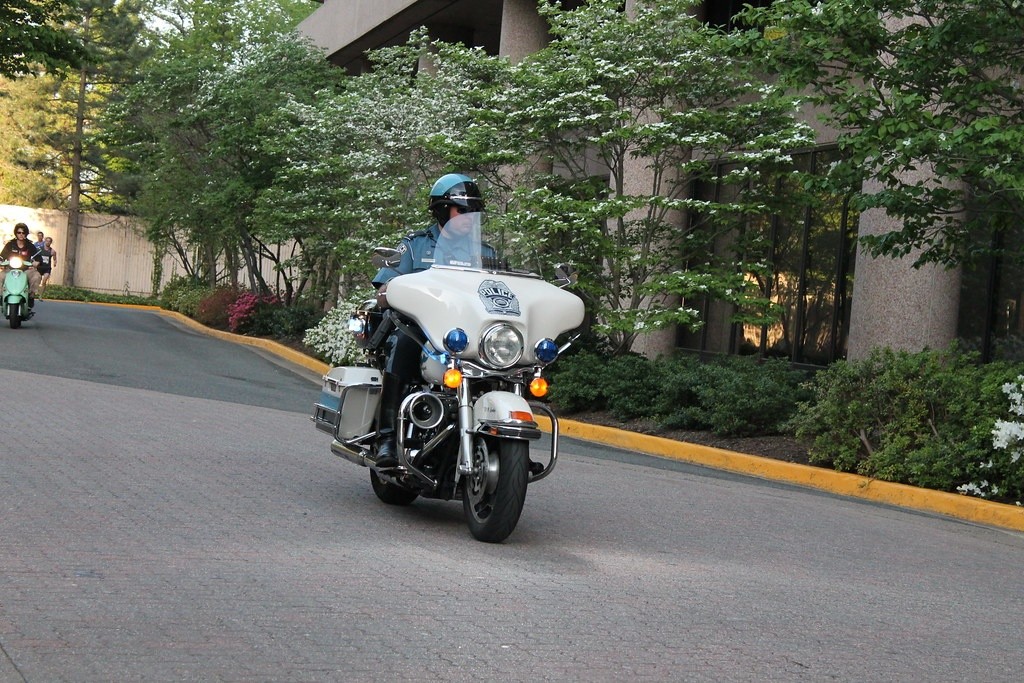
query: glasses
453 204 480 215
16 231 26 234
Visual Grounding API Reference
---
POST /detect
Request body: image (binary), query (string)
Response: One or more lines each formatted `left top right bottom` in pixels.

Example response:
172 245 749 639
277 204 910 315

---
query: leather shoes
529 457 544 475
374 439 399 469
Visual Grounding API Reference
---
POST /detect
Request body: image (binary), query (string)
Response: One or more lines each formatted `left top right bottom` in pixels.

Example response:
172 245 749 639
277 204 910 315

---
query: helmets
426 173 486 214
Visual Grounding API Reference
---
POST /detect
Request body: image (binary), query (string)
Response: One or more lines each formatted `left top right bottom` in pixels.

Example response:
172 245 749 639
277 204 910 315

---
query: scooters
0 243 45 330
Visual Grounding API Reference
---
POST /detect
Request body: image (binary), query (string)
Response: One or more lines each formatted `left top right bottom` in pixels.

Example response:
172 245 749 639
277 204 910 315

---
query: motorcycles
309 209 586 543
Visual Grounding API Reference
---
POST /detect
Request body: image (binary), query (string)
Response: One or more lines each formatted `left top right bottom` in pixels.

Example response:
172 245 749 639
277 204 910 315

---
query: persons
371 174 496 469
0 222 56 308
